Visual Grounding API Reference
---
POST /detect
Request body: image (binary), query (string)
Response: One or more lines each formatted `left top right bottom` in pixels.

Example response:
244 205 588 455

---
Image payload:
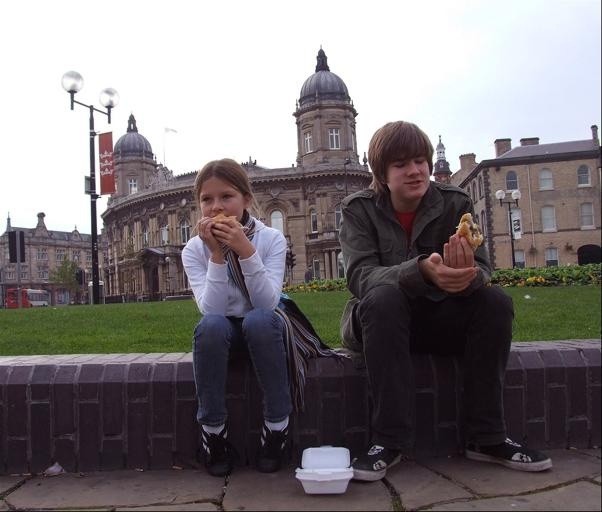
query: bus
6 289 49 308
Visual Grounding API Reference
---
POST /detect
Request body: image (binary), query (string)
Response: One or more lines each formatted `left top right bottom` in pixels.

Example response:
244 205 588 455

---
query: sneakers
350 436 404 482
198 422 233 477
464 433 553 473
256 417 291 474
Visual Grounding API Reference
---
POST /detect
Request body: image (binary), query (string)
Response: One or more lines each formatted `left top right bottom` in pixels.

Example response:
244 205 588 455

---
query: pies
213 213 239 223
457 212 484 252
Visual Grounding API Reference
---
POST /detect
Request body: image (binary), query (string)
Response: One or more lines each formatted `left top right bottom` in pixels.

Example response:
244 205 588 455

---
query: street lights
496 190 521 269
61 71 120 304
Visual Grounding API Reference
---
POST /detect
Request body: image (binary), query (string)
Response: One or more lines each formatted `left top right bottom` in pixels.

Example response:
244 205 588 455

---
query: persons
336 119 553 483
304 264 313 283
179 155 299 476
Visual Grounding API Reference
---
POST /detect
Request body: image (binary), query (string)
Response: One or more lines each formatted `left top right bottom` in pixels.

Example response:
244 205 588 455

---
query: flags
98 132 116 195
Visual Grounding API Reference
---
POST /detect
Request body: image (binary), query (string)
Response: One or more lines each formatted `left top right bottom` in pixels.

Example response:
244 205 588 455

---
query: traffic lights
286 249 296 268
